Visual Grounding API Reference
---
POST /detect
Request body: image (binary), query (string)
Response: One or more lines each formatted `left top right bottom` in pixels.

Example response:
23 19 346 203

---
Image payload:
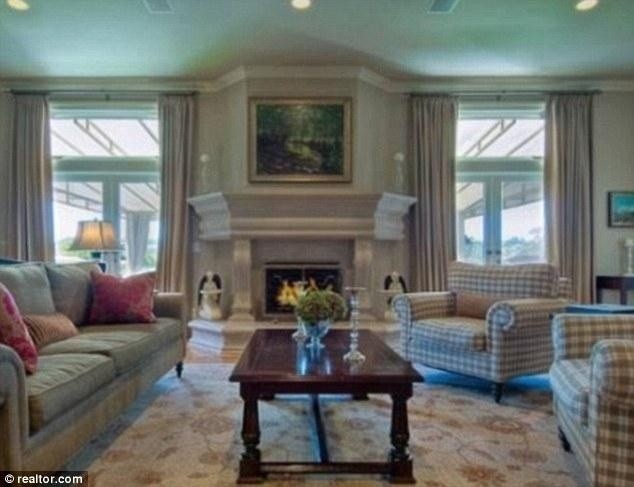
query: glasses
66 219 120 273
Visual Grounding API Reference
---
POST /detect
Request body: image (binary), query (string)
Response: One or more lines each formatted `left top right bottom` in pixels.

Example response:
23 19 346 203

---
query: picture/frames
597 275 634 305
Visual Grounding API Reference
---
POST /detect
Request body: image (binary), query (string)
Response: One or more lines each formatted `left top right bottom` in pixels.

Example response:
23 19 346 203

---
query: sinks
550 310 634 487
391 259 574 405
1 259 188 482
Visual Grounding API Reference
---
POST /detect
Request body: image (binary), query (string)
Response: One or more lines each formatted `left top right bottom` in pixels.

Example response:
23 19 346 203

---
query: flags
300 322 331 357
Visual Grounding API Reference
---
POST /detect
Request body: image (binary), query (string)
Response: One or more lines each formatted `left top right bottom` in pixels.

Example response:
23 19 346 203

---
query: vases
277 278 349 324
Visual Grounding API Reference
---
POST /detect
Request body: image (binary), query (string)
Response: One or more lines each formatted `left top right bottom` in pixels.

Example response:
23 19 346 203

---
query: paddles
136 364 590 485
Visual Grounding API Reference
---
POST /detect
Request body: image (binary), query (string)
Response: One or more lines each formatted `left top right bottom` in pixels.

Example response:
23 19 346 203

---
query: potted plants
609 190 634 231
248 95 354 182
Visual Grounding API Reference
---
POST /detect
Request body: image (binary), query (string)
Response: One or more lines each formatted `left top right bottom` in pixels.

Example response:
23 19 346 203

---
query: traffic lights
4 266 56 315
1 287 39 372
46 262 103 324
85 270 157 324
24 310 76 343
